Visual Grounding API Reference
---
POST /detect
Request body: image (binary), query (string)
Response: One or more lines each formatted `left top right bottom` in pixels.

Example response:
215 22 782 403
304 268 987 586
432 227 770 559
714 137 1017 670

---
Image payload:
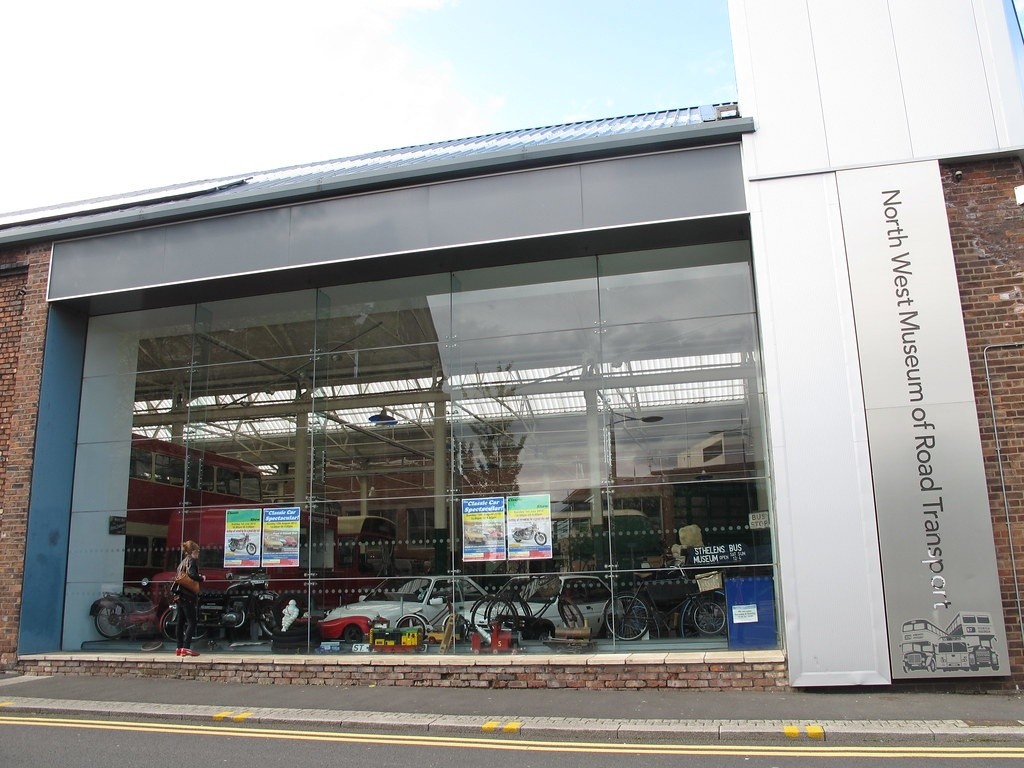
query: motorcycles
512 521 547 545
169 569 288 641
228 533 257 556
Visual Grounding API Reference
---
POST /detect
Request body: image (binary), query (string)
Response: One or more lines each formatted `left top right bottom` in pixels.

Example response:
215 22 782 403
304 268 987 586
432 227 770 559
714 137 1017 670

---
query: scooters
88 584 187 644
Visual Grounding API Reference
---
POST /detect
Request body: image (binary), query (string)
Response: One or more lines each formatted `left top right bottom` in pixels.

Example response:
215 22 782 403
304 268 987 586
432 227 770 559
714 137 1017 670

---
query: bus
336 515 397 605
123 431 264 596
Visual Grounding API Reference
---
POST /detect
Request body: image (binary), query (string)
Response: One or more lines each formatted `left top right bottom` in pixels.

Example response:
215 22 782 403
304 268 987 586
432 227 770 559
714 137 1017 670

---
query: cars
296 575 490 644
465 525 503 545
264 534 297 552
453 577 624 639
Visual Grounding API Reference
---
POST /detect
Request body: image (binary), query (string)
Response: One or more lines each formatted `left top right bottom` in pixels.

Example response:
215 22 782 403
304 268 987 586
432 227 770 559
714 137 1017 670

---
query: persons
171 540 208 657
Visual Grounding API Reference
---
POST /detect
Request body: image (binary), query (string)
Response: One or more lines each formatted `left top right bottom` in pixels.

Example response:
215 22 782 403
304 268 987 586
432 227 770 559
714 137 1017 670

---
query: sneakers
176 648 200 656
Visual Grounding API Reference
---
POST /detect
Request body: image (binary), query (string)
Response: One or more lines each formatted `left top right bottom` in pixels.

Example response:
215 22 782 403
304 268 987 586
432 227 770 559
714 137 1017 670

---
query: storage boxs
722 572 776 651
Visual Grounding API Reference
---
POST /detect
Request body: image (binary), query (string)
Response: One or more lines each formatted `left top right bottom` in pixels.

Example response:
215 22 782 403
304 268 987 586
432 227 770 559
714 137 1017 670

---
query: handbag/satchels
178 572 200 593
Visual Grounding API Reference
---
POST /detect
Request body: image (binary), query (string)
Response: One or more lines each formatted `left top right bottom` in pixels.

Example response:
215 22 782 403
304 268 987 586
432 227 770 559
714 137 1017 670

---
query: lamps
368 406 398 428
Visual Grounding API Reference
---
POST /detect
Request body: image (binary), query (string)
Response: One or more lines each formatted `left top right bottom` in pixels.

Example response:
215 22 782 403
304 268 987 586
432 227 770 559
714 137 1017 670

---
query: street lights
368 406 434 444
609 410 663 484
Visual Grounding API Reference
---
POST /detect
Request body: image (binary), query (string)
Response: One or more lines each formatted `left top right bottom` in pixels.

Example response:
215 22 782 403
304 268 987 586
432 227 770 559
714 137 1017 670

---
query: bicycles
469 572 584 645
601 557 726 641
483 580 576 637
395 590 469 642
622 592 726 636
